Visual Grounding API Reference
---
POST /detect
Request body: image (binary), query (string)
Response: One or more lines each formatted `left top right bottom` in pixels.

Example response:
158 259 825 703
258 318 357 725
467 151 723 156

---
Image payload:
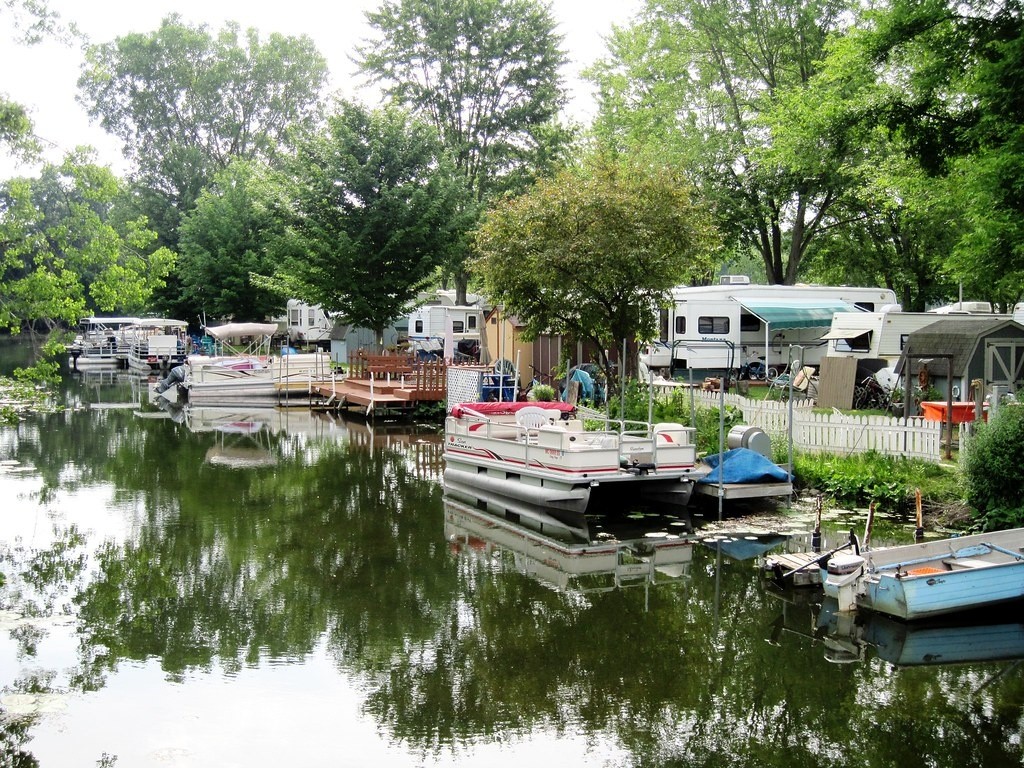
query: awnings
731 296 873 385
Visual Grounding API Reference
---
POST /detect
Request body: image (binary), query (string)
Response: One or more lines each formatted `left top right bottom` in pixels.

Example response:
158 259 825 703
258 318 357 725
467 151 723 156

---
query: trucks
285 290 504 355
640 274 901 381
819 304 1014 367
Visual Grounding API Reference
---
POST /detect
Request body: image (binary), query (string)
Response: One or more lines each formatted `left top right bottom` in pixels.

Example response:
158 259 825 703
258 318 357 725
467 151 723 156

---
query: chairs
514 406 551 443
780 365 816 392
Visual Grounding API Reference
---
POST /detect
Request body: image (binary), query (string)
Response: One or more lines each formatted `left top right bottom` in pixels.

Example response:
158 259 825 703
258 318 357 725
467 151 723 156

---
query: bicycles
524 364 554 400
739 348 768 381
855 377 893 409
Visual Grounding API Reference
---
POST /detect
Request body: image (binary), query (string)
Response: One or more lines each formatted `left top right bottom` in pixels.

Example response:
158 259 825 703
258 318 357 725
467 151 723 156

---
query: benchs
943 556 998 570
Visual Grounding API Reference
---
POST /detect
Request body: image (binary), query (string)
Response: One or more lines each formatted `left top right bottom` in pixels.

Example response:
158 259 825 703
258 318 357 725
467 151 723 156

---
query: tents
205 322 277 370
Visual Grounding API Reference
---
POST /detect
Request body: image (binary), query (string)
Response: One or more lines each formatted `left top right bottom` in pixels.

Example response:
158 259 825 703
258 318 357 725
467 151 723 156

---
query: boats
441 350 708 521
783 526 1024 622
69 358 346 469
61 315 335 402
440 486 705 615
784 593 1024 672
